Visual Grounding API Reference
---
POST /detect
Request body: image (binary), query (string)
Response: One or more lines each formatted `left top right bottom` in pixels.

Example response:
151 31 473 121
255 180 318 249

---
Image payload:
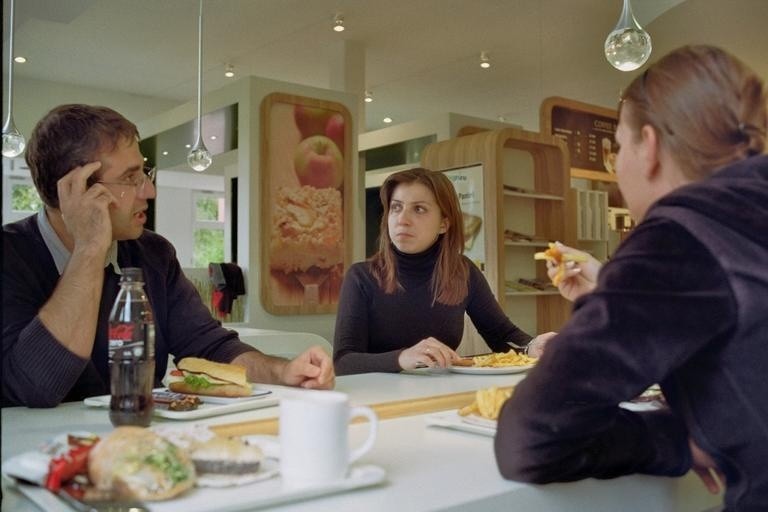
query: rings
424 346 430 353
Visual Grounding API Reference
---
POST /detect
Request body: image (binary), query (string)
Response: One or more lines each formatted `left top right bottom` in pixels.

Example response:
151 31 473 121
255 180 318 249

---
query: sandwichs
163 429 261 474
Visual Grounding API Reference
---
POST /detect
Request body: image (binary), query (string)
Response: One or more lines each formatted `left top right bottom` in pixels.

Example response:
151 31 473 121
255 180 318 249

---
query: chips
472 349 539 366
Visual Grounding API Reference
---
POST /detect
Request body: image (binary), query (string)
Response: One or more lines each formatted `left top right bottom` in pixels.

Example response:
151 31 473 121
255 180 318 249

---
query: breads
87 425 196 501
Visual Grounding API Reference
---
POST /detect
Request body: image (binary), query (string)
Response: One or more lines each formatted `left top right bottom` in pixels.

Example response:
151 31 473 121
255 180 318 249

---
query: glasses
91 163 158 190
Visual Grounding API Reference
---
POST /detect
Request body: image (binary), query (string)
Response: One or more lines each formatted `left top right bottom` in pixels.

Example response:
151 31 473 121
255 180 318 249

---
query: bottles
107 267 157 429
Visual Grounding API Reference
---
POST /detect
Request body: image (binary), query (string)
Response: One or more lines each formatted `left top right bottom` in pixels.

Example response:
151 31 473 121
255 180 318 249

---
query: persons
493 42 767 512
1 103 337 410
332 168 558 376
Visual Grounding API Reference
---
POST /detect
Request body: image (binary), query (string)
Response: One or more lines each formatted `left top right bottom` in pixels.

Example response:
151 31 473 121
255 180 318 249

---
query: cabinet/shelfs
421 130 571 337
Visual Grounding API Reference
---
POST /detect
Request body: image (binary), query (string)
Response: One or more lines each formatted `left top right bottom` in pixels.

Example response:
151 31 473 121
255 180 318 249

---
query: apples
294 105 345 189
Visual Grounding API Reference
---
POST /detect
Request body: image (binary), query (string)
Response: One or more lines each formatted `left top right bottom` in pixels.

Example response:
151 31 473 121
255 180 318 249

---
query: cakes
269 186 344 290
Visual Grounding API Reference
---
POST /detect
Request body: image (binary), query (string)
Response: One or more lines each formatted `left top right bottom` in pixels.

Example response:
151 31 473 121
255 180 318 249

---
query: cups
277 386 379 494
603 139 611 165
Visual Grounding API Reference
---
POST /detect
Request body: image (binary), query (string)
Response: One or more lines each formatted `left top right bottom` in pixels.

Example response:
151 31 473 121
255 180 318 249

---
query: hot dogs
167 357 253 397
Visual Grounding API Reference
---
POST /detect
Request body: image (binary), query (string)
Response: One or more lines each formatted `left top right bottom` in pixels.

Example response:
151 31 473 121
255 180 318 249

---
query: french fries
459 386 511 419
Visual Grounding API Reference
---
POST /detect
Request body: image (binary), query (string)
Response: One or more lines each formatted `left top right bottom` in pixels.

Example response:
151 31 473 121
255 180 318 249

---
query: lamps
186 0 213 173
2 1 26 157
604 0 653 72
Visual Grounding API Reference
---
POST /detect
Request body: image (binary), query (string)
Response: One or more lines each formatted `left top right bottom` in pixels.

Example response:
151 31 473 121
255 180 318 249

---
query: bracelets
525 338 534 355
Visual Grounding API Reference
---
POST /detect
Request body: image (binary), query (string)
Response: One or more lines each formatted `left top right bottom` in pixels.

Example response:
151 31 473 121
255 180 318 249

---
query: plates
446 364 531 375
457 410 498 434
603 157 613 174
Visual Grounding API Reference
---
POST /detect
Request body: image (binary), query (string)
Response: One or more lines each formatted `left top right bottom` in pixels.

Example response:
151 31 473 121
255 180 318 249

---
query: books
504 183 560 293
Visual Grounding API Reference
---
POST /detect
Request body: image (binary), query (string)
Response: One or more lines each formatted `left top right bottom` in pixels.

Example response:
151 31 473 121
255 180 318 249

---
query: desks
228 326 334 360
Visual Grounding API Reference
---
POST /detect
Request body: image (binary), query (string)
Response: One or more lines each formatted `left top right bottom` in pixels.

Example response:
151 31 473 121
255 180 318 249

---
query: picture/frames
261 92 353 317
